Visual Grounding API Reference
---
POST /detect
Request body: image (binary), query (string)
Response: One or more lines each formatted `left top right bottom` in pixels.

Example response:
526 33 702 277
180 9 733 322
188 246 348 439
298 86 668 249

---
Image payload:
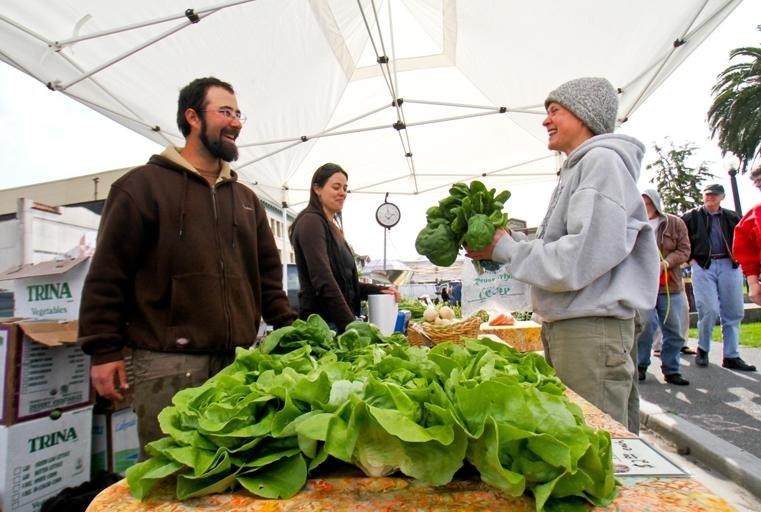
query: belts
706 254 734 260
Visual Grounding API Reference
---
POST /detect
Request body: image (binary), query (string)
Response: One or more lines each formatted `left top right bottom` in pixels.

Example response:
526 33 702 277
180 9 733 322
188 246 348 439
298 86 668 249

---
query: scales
370 192 411 286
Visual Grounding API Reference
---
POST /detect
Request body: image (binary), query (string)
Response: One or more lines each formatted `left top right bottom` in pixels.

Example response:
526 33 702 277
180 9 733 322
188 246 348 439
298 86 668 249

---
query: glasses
196 108 248 125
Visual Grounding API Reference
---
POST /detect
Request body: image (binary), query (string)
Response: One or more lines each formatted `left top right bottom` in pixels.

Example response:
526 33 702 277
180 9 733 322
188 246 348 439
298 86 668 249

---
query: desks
78 377 737 512
481 318 547 351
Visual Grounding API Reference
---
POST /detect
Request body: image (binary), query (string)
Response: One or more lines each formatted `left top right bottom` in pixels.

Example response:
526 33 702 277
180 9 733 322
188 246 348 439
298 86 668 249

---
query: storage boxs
0 247 145 512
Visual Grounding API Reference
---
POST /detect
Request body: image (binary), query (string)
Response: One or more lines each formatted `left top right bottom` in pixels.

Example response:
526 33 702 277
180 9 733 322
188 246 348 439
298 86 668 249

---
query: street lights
723 153 749 294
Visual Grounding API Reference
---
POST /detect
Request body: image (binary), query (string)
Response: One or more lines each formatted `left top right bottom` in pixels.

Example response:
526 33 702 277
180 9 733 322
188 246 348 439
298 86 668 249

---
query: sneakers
653 350 661 357
680 346 697 354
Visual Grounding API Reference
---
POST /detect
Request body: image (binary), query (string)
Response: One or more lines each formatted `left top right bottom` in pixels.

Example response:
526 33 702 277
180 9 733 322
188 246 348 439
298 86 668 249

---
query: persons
462 78 663 436
679 184 757 372
76 78 300 463
287 162 400 336
654 262 697 357
732 165 761 306
639 189 692 386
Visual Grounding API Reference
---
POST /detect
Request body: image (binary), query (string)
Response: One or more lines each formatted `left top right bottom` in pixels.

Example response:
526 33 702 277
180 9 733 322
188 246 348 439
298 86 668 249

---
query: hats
703 183 725 195
544 75 619 139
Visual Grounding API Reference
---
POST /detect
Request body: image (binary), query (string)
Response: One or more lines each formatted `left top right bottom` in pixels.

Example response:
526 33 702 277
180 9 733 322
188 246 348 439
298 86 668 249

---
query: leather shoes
722 354 757 372
637 361 648 383
694 346 709 368
662 373 691 386
